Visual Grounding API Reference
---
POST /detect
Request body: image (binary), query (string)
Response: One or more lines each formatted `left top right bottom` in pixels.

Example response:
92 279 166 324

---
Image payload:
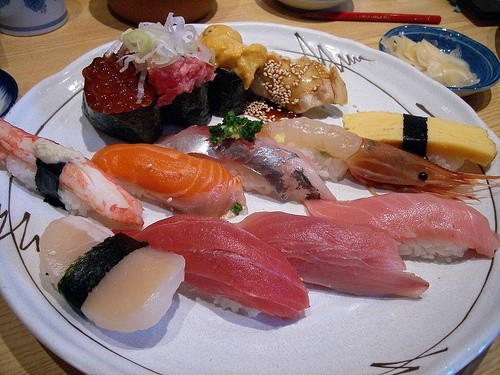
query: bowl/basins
379 25 500 97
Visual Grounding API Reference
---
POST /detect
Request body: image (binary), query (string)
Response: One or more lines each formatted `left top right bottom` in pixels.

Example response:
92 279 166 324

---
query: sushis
0 12 500 335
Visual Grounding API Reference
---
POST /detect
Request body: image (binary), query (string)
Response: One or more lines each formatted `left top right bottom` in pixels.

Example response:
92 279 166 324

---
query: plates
0 23 500 375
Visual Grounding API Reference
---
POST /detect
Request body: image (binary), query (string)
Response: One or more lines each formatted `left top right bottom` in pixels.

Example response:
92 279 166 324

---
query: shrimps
255 118 500 205
0 116 147 229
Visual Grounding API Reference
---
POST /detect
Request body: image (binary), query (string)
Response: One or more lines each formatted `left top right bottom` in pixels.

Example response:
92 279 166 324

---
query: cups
107 0 216 30
0 0 69 36
